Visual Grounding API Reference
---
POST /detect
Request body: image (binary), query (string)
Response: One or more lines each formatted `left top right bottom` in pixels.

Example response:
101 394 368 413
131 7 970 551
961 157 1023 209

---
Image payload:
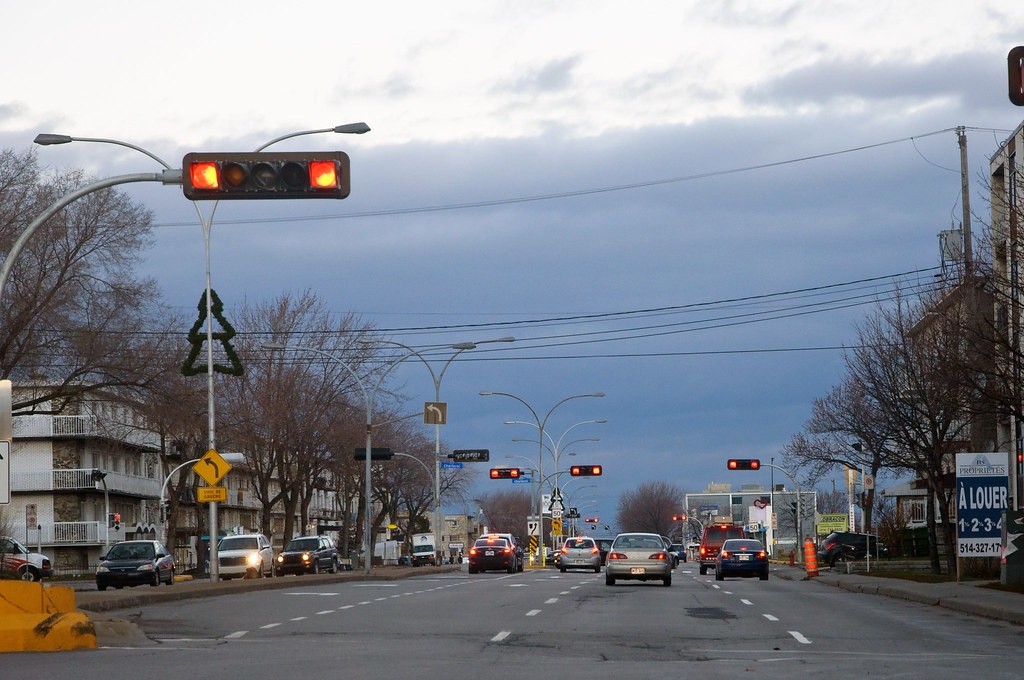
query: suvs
276 534 339 574
818 529 889 567
217 534 276 580
0 535 54 582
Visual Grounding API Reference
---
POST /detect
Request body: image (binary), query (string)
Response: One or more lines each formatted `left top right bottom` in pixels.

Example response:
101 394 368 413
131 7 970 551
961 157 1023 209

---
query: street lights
33 122 371 583
258 341 476 570
359 336 516 566
851 442 865 534
479 391 608 569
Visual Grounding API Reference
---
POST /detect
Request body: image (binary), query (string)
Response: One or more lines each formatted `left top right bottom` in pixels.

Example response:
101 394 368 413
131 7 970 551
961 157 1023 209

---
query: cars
697 522 769 580
545 536 614 573
468 530 525 574
96 539 176 591
605 533 687 587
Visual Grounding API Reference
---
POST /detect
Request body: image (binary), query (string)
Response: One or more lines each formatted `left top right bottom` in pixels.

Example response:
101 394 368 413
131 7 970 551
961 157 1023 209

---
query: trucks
412 532 437 567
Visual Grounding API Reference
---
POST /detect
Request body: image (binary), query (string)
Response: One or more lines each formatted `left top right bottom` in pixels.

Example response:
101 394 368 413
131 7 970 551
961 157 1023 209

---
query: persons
458 555 462 564
805 534 812 541
450 555 454 564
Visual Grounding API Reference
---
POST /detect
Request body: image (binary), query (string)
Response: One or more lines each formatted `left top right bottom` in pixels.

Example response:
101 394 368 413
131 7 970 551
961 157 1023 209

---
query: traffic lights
673 515 686 521
570 464 603 477
114 514 120 532
182 151 351 201
490 467 520 479
727 458 761 471
586 517 598 522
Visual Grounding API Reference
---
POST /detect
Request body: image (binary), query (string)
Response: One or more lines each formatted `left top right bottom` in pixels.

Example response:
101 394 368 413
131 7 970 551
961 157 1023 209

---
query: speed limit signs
749 524 759 533
552 508 562 517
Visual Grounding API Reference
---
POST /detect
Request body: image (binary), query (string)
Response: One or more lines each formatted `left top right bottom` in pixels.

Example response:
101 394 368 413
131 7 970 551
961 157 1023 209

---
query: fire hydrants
788 551 795 566
